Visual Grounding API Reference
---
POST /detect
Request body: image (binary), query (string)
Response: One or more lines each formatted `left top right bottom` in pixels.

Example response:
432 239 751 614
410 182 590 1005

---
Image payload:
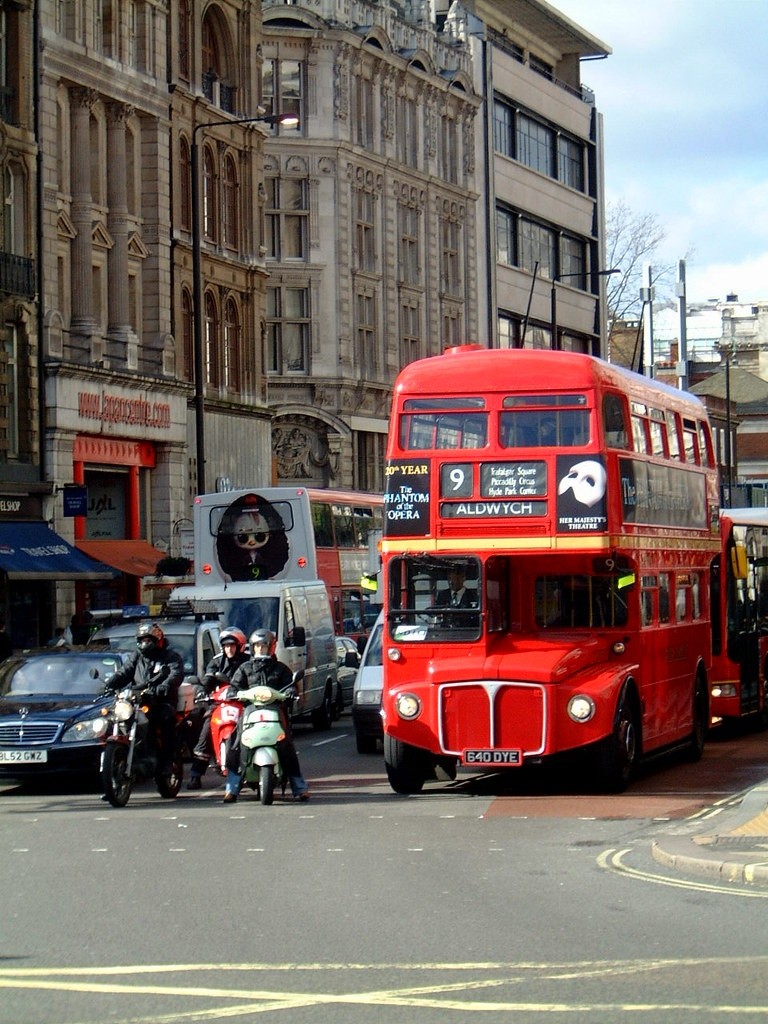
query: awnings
0 522 120 582
74 538 196 591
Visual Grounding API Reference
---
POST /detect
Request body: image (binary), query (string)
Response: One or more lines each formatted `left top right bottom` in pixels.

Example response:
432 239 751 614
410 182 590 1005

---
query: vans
159 578 339 732
352 598 448 755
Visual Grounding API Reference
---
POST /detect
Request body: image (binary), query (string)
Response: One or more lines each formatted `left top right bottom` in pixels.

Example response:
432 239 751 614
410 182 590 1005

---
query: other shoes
300 791 309 803
187 776 202 790
223 793 237 802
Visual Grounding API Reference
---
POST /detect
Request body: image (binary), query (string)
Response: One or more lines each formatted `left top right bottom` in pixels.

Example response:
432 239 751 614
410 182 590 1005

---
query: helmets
249 629 276 657
219 627 245 654
136 623 164 658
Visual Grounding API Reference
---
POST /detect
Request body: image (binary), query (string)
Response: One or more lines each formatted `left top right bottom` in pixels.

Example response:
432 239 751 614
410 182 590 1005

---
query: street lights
713 340 735 508
191 112 301 495
550 268 622 350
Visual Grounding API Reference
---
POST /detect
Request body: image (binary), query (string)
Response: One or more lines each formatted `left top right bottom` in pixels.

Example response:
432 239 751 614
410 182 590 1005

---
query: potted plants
153 555 192 582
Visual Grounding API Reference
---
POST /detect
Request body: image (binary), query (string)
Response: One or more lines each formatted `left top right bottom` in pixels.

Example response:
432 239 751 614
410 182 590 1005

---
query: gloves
228 691 237 700
284 690 294 700
196 692 208 700
97 685 107 695
155 687 165 696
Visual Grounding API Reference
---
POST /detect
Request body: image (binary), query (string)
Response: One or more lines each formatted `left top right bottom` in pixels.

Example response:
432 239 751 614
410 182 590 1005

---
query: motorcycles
88 668 192 808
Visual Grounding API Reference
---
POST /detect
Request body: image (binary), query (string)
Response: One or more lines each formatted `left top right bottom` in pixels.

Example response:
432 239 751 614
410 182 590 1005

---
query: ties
452 592 458 607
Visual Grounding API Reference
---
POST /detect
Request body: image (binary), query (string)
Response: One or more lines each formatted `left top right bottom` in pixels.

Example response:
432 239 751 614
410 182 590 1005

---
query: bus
368 341 732 798
194 488 388 656
710 505 768 728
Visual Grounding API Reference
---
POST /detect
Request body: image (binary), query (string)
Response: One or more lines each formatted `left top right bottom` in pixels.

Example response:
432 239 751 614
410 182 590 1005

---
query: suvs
85 606 225 753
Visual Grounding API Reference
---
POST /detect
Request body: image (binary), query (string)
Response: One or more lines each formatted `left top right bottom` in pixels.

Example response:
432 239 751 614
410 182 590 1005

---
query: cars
0 645 139 790
335 637 362 722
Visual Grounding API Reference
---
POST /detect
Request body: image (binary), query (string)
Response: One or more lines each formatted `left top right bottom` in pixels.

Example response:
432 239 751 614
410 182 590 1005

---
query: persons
545 575 600 628
0 625 13 663
186 627 250 790
434 567 481 628
97 624 185 801
535 416 574 447
47 610 103 649
289 427 312 477
218 630 309 803
272 428 285 455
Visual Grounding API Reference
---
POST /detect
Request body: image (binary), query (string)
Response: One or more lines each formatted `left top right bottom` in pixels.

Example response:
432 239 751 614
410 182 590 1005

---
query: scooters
214 668 306 805
186 675 250 790
